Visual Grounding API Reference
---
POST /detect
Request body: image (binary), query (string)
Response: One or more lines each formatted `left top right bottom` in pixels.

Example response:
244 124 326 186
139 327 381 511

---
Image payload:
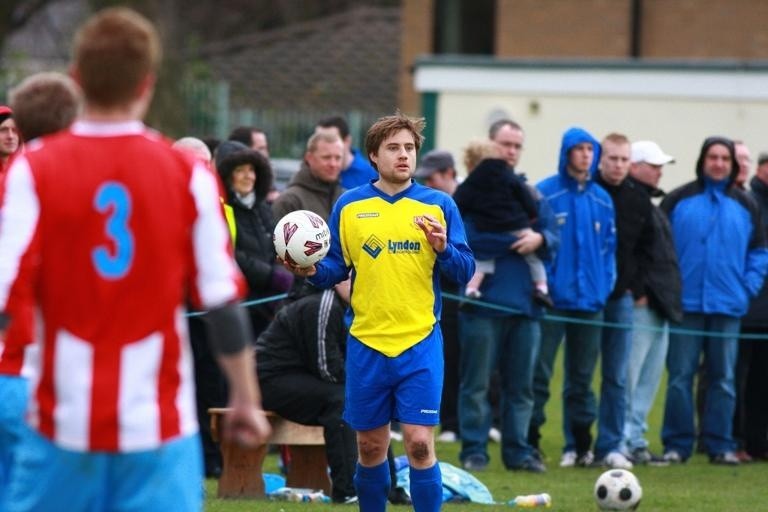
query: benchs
206 406 333 496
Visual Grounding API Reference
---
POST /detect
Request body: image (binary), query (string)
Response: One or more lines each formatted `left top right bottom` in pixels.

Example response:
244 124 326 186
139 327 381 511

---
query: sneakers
645 459 669 468
663 452 684 464
607 453 631 470
560 452 578 468
709 451 738 466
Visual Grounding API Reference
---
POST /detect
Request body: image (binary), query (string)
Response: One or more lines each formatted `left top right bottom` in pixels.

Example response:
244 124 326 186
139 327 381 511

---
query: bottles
514 492 553 507
292 493 332 503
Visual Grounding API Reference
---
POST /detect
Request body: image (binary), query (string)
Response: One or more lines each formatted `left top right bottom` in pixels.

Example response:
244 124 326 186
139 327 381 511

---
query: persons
171 137 236 261
311 117 380 188
557 134 657 468
620 139 683 468
1 9 270 512
272 109 476 511
525 128 617 468
2 105 22 156
227 126 271 195
253 263 411 505
411 150 460 198
698 141 768 464
0 72 79 214
751 156 768 200
656 136 767 466
271 130 347 254
446 118 561 470
189 141 292 479
452 141 558 310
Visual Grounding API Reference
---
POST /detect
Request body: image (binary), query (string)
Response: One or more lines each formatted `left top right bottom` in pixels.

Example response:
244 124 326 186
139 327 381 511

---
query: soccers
593 469 643 512
273 210 332 269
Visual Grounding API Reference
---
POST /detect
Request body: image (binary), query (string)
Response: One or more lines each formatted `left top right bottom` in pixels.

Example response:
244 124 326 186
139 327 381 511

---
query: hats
630 139 676 169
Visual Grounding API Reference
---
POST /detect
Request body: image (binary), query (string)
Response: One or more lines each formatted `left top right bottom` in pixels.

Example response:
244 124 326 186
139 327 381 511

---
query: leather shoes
513 458 547 473
465 456 487 470
